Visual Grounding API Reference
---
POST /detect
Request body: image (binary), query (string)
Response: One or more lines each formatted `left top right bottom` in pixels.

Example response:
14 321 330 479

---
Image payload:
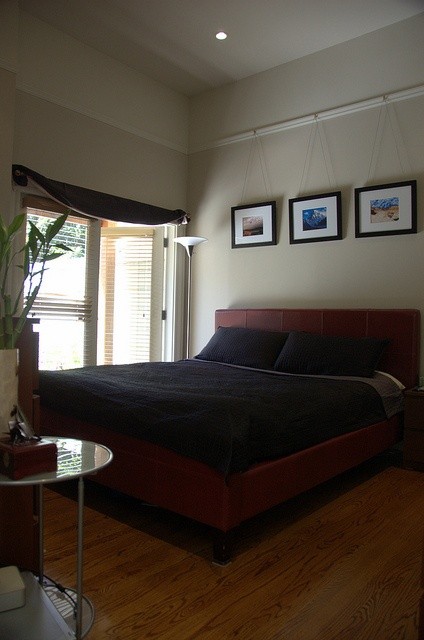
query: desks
0 437 113 640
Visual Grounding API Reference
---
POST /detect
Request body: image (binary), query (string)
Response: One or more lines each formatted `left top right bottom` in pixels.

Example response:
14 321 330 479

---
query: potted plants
0 211 72 438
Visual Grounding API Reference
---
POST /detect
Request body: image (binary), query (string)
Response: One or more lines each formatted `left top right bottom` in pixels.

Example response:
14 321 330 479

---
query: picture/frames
288 191 342 244
354 181 417 237
231 200 277 248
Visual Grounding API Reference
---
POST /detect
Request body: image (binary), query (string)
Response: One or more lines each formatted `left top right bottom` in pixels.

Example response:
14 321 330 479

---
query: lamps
173 237 207 359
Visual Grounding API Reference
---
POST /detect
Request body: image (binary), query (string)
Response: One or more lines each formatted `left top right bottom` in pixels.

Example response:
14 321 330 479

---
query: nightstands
397 385 424 473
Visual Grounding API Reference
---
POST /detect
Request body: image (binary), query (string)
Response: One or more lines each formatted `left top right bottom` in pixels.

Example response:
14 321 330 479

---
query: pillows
194 325 289 371
274 330 383 378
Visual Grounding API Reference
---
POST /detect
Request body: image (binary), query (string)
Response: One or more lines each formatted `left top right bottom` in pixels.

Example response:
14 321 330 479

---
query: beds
37 309 420 564
